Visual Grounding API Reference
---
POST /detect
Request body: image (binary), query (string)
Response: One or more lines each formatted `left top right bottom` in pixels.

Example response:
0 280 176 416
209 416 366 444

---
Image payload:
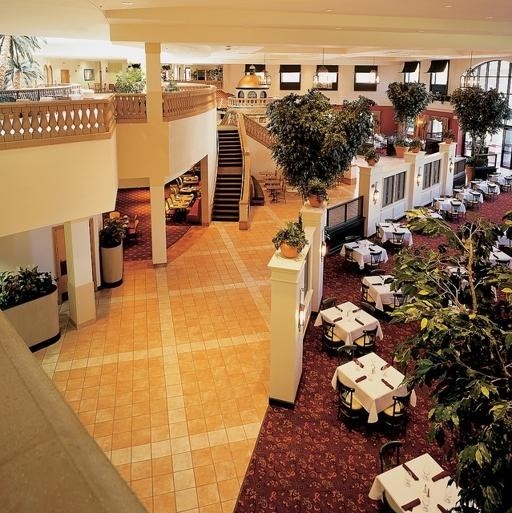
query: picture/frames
84 70 95 81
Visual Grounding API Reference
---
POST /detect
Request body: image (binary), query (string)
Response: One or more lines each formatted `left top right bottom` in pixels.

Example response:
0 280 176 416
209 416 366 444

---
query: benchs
187 196 202 221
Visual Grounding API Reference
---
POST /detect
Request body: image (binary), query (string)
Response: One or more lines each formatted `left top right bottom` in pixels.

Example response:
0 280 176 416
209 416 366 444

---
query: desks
68 87 95 100
368 452 480 513
259 171 281 204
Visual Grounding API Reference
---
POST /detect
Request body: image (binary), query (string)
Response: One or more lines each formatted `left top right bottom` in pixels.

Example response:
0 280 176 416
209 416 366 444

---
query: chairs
360 268 411 324
274 170 287 204
379 441 403 473
414 171 512 239
165 175 183 225
88 82 116 93
390 350 407 373
337 345 357 366
336 375 365 432
383 391 412 437
376 218 413 256
313 297 384 359
339 235 389 275
109 209 139 244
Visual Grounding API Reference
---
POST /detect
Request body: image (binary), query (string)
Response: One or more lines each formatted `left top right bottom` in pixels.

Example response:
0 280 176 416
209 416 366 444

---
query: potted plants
295 176 330 208
443 128 456 144
0 265 62 352
272 220 310 258
465 156 476 181
99 213 129 287
393 139 410 158
409 139 424 154
362 145 380 166
